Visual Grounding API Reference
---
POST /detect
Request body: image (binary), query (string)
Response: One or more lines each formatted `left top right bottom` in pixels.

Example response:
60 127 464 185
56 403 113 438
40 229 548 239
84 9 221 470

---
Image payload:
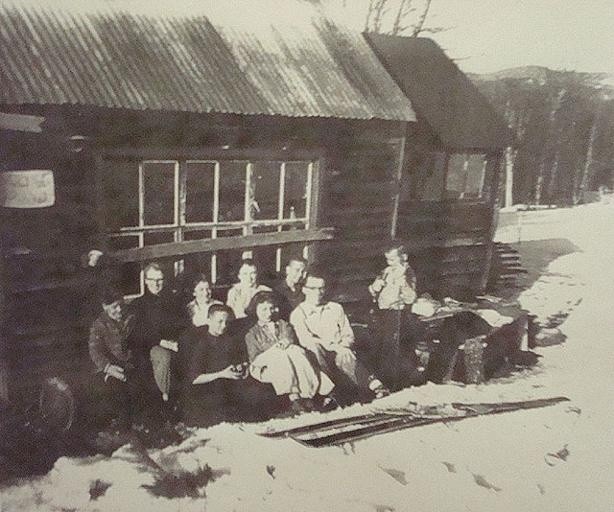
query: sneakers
372 383 390 400
